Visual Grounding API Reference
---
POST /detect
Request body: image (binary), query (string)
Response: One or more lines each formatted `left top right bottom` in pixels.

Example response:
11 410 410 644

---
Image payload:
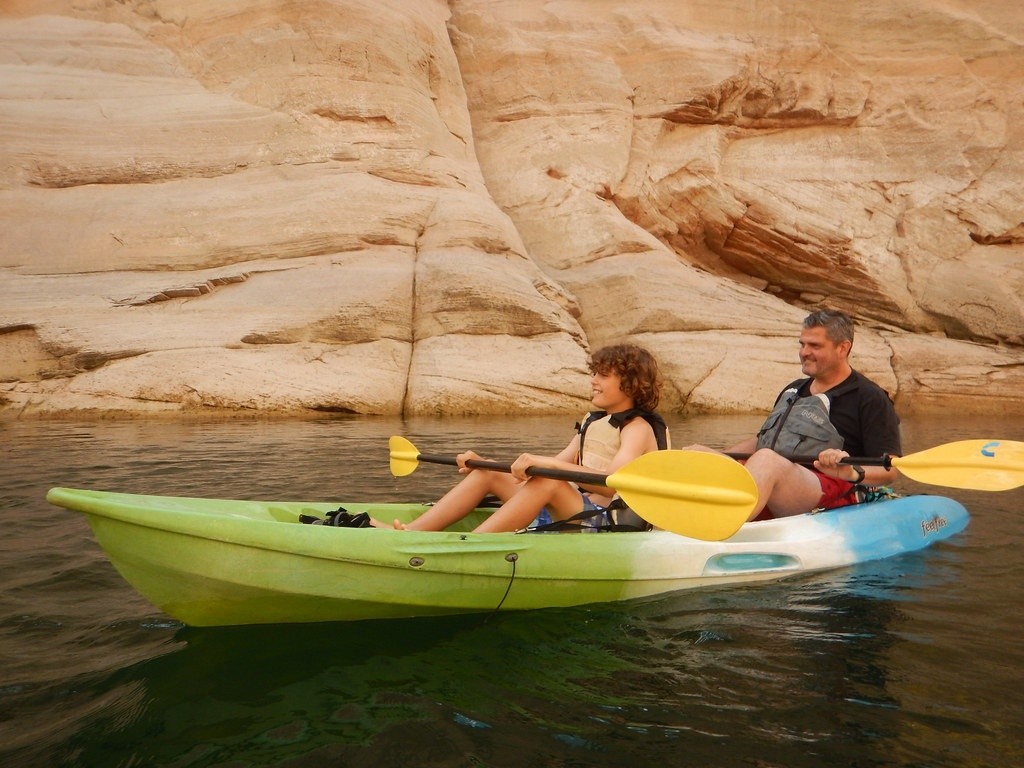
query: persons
682 309 903 522
356 343 671 533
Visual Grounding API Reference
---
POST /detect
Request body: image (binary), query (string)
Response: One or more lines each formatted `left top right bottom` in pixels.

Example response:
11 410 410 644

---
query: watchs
846 464 865 483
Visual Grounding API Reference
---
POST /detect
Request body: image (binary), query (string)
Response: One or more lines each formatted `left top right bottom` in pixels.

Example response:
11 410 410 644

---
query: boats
45 482 972 629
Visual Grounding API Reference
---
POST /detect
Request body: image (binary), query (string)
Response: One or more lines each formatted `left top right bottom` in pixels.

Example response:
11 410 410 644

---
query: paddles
723 439 1024 491
389 435 759 542
